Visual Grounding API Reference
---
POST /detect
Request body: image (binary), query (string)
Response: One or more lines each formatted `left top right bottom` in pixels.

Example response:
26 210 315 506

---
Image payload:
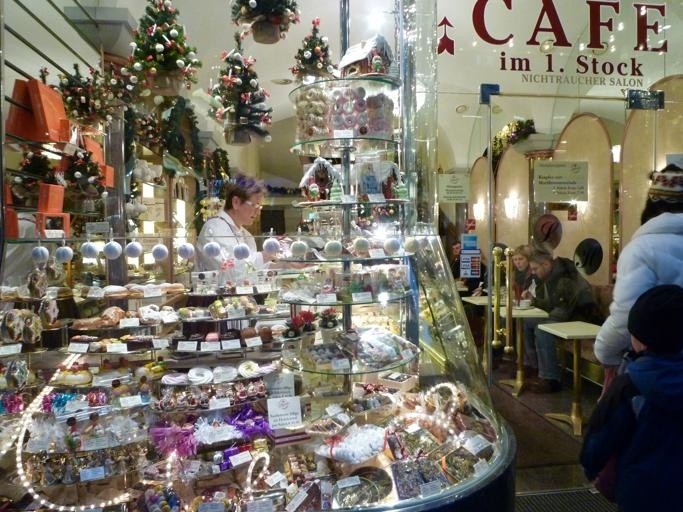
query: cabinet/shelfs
2 233 518 509
281 74 420 395
122 121 197 310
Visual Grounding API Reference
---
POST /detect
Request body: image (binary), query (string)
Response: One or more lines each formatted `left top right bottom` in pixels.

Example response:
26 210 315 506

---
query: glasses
246 201 263 210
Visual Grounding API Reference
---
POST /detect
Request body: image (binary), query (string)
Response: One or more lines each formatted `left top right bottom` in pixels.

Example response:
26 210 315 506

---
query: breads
0 282 285 352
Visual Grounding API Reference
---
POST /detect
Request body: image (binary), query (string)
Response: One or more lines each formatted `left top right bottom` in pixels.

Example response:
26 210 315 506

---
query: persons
472 243 508 357
579 284 683 512
523 250 603 394
592 163 683 370
183 173 304 339
512 244 536 380
450 242 486 317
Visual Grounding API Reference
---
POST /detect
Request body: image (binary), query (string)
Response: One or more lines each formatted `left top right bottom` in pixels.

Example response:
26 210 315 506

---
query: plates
336 466 391 507
512 306 535 310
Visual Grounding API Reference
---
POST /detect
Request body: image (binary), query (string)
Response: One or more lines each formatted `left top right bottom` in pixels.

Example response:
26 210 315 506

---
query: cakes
54 356 171 386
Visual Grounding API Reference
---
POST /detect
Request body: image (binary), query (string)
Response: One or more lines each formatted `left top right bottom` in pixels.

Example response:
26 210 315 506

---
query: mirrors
0 0 110 292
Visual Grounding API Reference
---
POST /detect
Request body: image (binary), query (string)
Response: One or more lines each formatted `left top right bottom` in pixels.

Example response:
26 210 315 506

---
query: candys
293 87 394 138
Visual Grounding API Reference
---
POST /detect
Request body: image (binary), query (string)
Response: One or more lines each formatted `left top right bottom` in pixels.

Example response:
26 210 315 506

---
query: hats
628 284 683 349
640 163 683 224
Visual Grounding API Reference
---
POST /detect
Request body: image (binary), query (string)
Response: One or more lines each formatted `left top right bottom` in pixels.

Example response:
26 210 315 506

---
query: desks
451 281 606 439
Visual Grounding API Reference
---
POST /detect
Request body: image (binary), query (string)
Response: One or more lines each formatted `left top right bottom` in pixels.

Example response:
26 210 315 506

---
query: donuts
161 360 260 387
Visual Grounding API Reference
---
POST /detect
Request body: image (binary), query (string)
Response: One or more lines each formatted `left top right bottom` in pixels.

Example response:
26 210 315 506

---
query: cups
518 300 531 307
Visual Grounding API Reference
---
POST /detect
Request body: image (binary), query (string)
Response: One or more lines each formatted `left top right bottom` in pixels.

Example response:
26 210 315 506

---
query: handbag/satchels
594 368 616 502
